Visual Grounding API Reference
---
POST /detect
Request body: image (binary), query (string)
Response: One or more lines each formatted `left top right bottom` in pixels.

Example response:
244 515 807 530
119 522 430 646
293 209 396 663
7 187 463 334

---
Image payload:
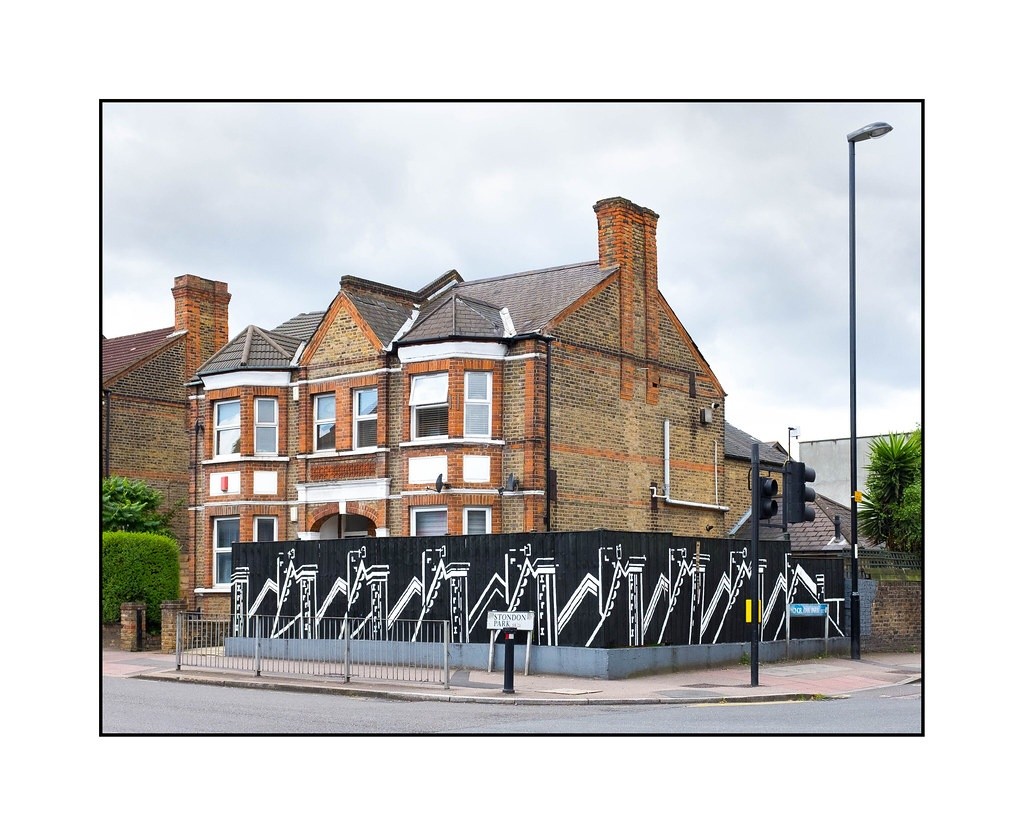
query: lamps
707 525 713 530
436 474 450 492
195 420 204 434
507 473 519 492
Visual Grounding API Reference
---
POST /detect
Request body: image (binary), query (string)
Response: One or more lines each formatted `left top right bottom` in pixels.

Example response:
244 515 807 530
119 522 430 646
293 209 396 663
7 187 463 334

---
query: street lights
788 427 796 459
848 121 893 660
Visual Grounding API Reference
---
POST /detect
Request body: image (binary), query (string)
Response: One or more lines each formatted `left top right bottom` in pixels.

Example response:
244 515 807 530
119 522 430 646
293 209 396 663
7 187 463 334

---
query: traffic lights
783 459 815 523
759 476 778 520
